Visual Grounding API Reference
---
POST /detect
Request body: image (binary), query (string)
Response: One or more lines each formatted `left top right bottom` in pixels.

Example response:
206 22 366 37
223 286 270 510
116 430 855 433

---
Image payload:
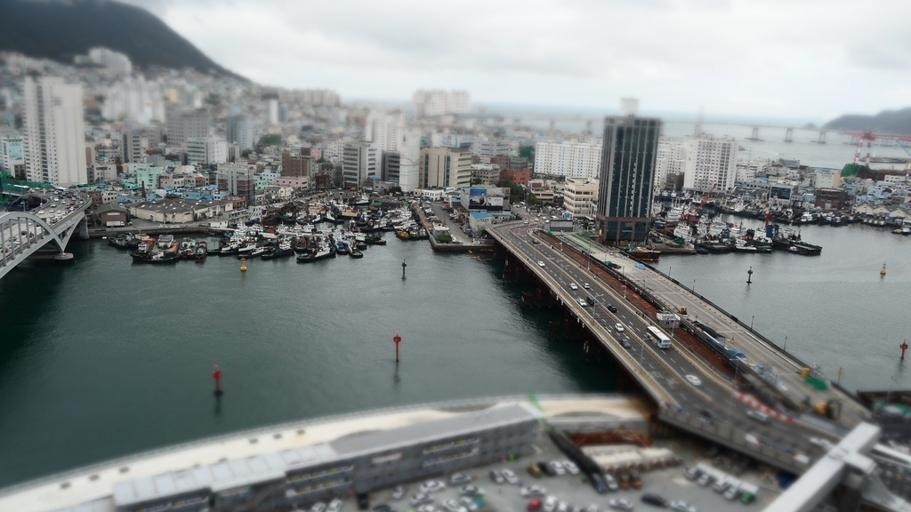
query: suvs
745 409 769 423
607 304 616 312
614 323 624 333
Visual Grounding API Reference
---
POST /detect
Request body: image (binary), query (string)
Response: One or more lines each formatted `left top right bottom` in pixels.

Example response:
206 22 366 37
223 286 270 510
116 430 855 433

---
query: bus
645 325 671 349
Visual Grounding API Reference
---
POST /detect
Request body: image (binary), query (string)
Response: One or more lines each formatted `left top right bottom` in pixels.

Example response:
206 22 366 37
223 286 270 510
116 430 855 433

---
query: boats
653 187 911 256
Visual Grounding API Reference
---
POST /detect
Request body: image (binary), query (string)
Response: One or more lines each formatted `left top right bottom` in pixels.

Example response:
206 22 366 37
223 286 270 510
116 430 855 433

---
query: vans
790 450 810 466
744 434 760 448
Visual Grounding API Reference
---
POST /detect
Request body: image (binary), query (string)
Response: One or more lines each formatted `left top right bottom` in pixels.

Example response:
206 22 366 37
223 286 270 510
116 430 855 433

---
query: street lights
692 279 695 292
728 358 739 381
782 335 788 354
591 294 606 327
750 314 754 330
553 230 646 301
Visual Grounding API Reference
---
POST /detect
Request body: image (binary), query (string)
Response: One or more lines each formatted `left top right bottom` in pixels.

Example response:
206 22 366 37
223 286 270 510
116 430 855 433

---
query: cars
0 181 89 268
585 295 596 306
576 297 587 307
619 338 632 349
532 239 538 244
538 260 545 266
696 405 718 422
685 374 702 387
511 201 559 226
810 437 830 448
